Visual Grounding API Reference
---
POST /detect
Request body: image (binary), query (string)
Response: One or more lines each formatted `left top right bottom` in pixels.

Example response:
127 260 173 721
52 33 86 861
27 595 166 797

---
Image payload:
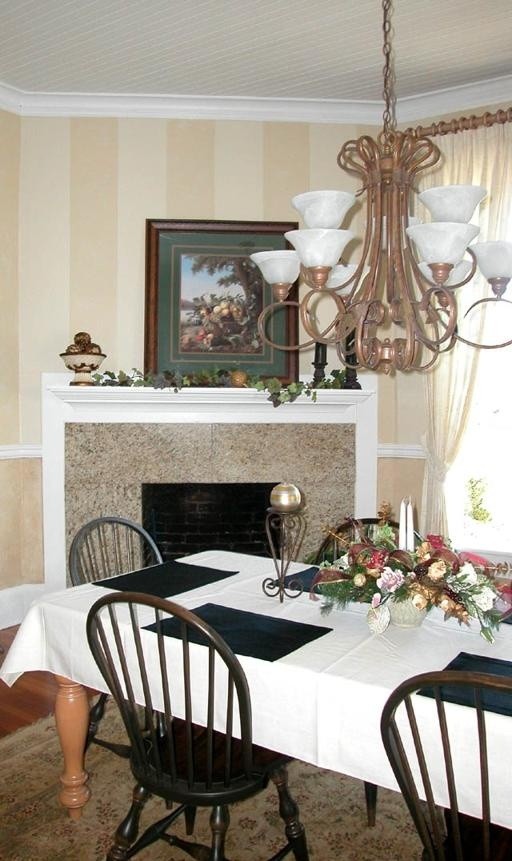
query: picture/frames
144 218 299 387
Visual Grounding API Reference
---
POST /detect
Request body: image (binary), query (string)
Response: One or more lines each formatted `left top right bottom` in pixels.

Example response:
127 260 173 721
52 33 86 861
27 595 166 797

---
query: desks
0 550 512 831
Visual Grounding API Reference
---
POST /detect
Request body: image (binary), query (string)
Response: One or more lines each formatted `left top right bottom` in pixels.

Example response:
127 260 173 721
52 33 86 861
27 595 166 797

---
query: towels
141 602 334 662
269 566 324 595
91 559 240 599
416 652 512 716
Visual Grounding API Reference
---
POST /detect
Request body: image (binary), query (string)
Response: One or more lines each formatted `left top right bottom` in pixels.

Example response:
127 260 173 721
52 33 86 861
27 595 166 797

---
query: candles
345 327 358 366
399 497 406 551
406 495 415 553
315 342 327 364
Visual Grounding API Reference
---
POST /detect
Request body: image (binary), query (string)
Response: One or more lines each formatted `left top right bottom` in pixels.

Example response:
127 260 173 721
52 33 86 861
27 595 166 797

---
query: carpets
0 693 447 861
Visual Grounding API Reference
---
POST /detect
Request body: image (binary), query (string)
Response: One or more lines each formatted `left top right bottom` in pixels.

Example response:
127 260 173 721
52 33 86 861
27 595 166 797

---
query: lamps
262 483 305 603
249 0 512 376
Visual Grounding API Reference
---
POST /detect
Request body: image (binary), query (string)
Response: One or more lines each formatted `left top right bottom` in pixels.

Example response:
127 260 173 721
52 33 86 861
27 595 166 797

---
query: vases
381 593 430 628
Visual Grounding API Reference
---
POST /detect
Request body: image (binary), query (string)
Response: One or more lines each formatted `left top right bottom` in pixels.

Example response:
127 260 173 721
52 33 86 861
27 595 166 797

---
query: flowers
309 511 512 645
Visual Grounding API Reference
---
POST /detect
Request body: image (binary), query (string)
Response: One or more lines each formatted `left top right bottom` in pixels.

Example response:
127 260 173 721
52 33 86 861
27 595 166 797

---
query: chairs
69 517 173 810
86 591 309 861
380 670 511 861
315 518 426 826
